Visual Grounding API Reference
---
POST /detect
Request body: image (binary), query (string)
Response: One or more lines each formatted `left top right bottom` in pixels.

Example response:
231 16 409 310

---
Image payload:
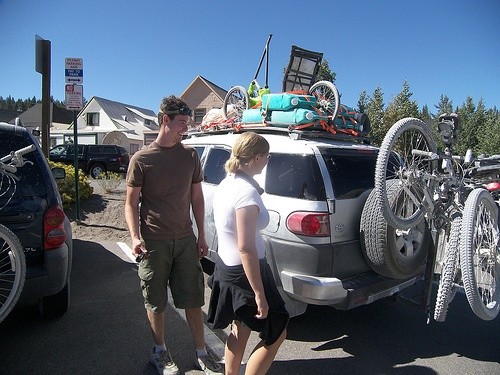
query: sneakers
150 346 180 375
194 351 224 375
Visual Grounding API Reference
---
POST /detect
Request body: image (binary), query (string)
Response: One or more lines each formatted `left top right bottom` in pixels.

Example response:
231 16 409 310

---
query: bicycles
0 144 35 323
375 112 500 321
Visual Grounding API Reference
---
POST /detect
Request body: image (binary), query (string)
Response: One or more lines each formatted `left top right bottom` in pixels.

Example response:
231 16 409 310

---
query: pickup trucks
49 143 129 178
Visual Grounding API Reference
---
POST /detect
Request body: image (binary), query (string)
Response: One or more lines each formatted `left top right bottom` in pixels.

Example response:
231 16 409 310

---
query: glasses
159 106 192 117
258 154 271 160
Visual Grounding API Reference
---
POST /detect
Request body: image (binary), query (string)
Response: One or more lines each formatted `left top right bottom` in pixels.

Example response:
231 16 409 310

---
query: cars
465 155 500 202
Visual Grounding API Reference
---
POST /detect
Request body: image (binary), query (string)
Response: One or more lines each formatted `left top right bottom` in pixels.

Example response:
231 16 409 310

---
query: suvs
178 133 433 317
0 117 73 301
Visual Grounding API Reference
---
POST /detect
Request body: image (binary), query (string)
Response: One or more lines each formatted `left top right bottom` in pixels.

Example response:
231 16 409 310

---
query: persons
206 132 290 375
124 95 225 375
